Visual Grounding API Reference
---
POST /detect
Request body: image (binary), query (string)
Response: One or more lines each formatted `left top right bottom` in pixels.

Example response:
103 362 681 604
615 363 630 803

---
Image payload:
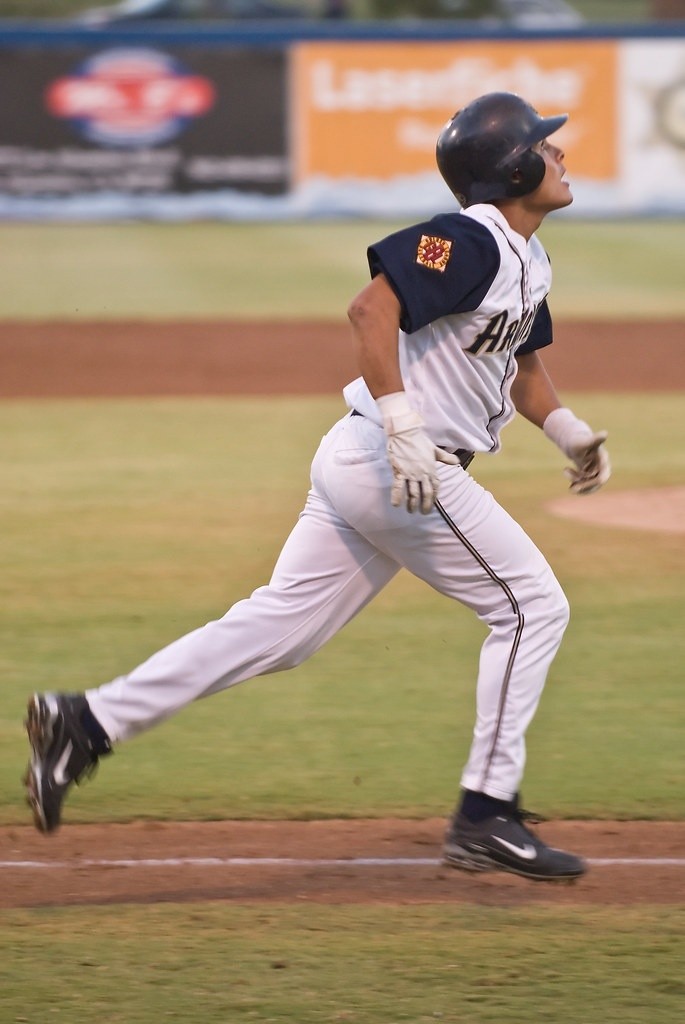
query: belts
351 408 476 472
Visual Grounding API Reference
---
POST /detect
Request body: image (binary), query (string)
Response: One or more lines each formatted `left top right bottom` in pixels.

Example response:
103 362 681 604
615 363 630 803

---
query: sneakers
441 804 585 879
23 690 114 836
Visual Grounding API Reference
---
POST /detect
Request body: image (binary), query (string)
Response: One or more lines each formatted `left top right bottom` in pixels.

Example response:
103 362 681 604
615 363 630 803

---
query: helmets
435 91 572 209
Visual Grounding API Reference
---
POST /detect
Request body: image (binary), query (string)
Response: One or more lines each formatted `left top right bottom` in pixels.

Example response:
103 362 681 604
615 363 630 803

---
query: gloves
374 391 461 517
543 410 609 497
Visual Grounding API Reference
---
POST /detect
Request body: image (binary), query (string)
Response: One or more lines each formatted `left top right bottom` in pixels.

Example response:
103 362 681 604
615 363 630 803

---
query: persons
24 92 610 882
